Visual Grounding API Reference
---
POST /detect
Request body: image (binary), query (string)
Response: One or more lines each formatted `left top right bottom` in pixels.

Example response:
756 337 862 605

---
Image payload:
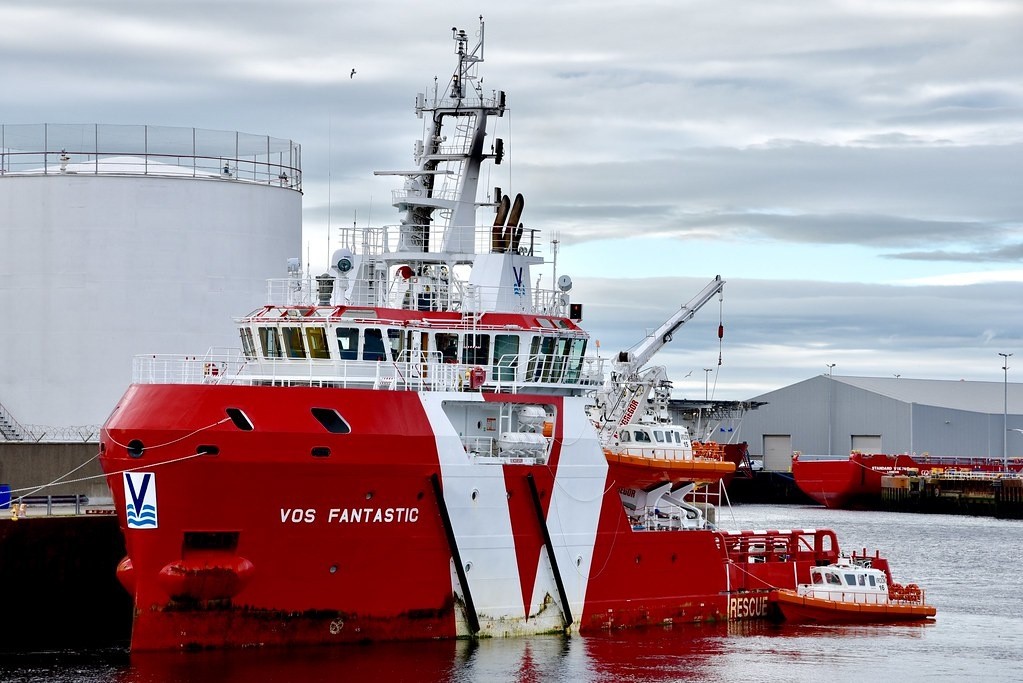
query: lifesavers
202 363 218 378
920 470 929 476
888 584 921 601
691 441 719 458
470 367 485 389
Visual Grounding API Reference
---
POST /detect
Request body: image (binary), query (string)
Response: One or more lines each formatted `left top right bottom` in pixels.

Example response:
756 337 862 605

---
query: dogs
10 503 27 516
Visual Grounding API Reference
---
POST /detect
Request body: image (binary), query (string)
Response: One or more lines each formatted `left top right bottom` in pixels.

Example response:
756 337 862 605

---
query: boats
98 15 893 654
792 449 1023 511
769 549 936 625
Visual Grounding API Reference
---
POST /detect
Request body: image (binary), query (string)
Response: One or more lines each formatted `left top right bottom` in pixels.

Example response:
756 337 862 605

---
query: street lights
826 363 836 455
999 353 1014 467
704 369 713 400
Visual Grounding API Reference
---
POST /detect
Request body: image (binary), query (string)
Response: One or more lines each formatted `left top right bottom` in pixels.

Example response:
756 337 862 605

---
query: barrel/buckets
0 483 12 509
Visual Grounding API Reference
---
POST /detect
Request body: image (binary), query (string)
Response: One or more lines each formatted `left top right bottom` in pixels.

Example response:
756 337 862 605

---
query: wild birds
350 68 357 79
